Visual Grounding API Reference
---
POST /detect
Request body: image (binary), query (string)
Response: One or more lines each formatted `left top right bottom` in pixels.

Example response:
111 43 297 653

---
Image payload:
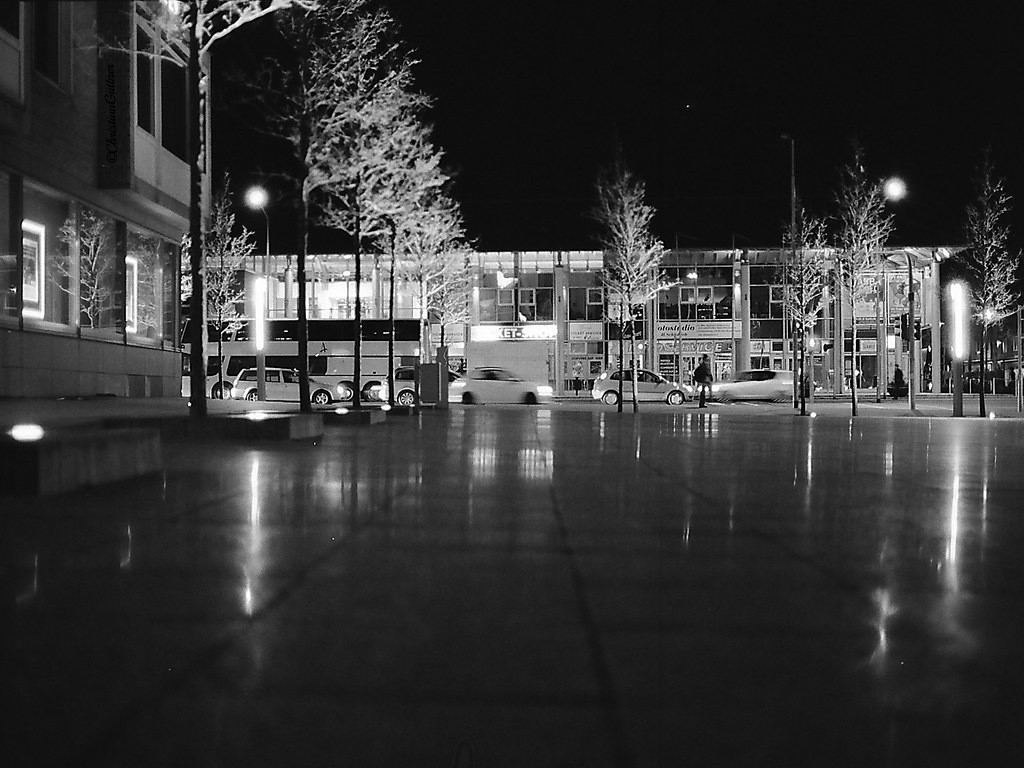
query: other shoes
892 397 898 400
699 404 707 408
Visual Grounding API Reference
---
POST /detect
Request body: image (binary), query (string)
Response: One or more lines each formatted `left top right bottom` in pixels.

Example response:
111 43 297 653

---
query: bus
181 319 426 400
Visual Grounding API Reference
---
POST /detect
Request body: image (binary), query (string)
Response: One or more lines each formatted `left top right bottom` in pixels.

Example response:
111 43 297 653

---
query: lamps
586 265 591 271
536 266 539 271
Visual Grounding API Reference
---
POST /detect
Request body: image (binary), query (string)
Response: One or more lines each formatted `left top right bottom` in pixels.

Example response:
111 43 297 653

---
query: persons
892 364 903 400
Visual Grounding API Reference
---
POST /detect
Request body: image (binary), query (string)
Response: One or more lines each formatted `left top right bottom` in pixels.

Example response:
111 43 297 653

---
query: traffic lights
914 320 921 340
894 315 906 338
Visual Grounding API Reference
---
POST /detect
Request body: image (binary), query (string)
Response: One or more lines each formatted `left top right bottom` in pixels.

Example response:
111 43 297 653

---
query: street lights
876 179 907 403
246 187 270 319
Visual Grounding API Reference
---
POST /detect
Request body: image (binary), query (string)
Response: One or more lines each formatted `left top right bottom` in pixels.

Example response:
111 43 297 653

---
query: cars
379 365 467 408
231 367 347 405
697 369 801 404
448 366 553 406
592 367 695 406
